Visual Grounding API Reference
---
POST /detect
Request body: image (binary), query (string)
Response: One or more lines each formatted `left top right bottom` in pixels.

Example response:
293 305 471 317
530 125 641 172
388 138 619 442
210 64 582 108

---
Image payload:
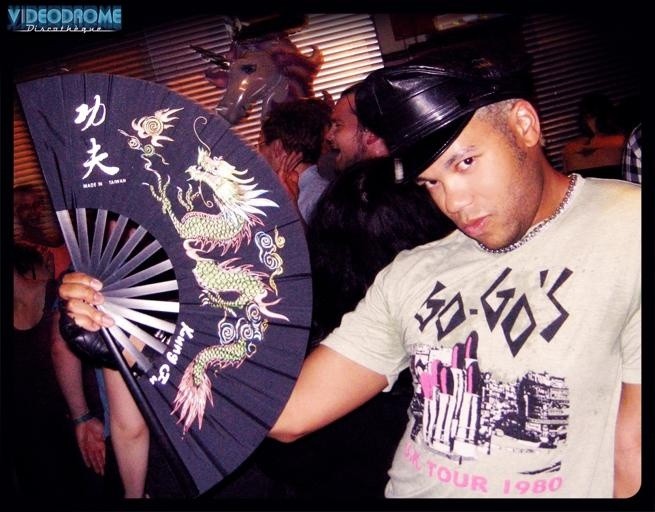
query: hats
355 39 530 182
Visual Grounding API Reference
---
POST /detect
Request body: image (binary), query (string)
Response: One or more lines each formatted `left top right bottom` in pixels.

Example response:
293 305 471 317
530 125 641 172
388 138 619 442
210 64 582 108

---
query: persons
15 56 640 499
